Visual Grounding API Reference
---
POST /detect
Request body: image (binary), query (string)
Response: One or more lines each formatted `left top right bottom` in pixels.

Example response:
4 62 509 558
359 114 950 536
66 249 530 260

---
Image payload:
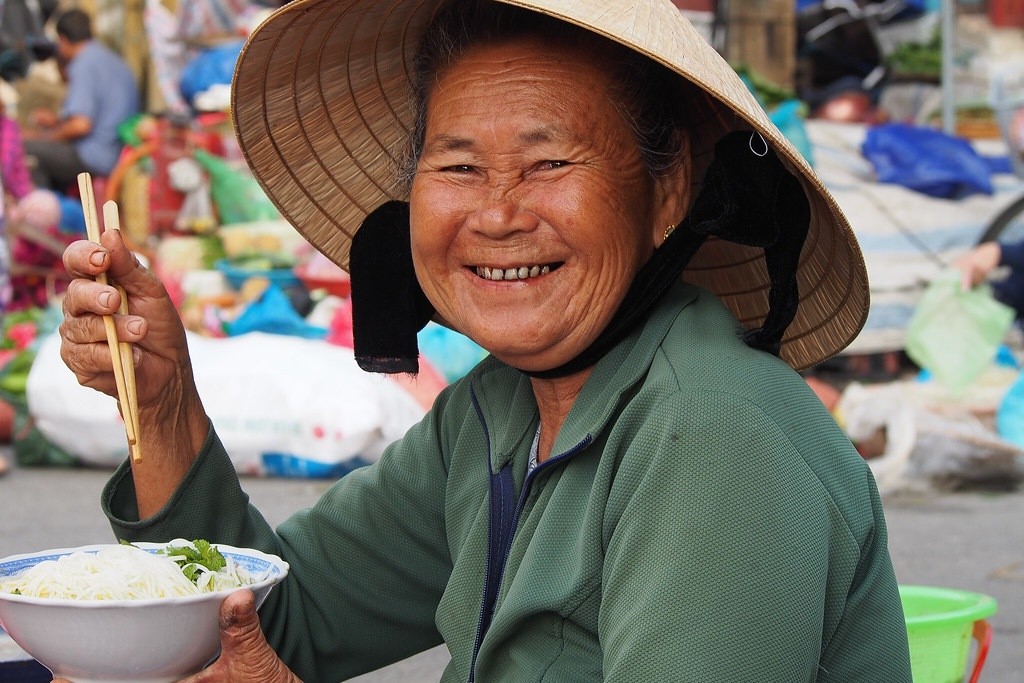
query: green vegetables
119 538 225 591
10 588 21 594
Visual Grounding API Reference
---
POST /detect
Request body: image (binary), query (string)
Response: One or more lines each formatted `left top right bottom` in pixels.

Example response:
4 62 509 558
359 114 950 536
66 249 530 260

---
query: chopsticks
76 172 142 463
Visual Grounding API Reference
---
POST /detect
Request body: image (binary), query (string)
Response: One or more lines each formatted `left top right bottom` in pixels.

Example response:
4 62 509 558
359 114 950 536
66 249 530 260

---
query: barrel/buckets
898 584 997 682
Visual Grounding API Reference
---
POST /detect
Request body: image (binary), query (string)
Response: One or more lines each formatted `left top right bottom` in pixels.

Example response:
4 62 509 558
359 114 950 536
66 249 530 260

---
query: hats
230 0 870 372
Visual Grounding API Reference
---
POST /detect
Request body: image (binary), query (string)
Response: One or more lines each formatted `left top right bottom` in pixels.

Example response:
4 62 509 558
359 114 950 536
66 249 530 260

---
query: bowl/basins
0 542 289 682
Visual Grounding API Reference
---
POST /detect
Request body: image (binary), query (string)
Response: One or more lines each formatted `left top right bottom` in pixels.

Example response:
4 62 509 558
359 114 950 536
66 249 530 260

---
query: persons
58 0 913 683
952 58 1024 332
0 0 270 313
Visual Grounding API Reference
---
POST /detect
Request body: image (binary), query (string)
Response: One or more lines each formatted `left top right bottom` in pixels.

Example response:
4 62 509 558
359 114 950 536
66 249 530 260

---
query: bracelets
158 76 179 89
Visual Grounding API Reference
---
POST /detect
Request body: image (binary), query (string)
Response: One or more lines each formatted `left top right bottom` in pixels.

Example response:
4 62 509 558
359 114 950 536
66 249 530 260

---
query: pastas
0 545 273 602
